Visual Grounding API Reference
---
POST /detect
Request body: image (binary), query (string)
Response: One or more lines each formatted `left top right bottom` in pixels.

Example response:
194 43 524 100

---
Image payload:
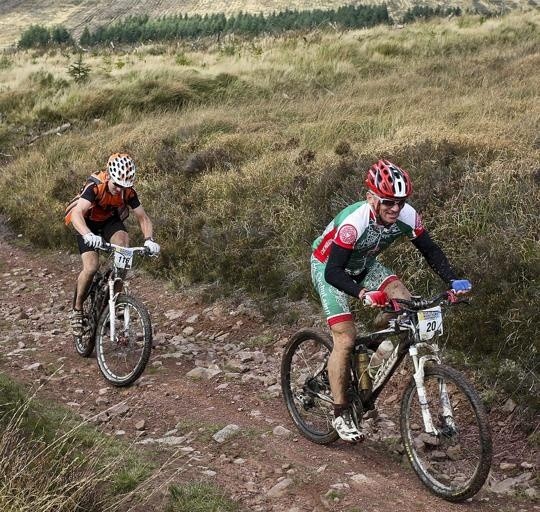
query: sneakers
71 307 84 337
332 410 365 443
115 306 125 318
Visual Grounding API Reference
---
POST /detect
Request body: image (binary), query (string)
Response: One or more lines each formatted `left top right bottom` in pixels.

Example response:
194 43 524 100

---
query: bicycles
280 288 492 503
73 236 161 386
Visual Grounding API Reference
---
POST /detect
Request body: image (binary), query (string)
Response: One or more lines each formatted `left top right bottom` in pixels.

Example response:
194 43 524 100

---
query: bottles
357 345 370 390
368 340 395 379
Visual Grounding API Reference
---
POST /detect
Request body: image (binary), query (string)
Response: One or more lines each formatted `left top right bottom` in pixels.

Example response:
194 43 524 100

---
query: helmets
365 159 413 198
107 154 136 188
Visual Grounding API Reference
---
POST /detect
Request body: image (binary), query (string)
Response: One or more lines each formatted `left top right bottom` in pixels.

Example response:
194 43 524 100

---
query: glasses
374 194 405 209
112 181 128 190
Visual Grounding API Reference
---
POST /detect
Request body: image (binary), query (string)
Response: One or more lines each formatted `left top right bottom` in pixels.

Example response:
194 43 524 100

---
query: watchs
141 235 156 242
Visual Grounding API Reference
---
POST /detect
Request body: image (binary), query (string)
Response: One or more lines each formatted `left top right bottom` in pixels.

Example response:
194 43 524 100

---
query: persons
64 154 160 336
310 160 473 445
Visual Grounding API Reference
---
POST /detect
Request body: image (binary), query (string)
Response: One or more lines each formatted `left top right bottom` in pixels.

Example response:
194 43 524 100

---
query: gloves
360 290 390 308
450 279 472 296
82 232 111 251
143 237 161 255
